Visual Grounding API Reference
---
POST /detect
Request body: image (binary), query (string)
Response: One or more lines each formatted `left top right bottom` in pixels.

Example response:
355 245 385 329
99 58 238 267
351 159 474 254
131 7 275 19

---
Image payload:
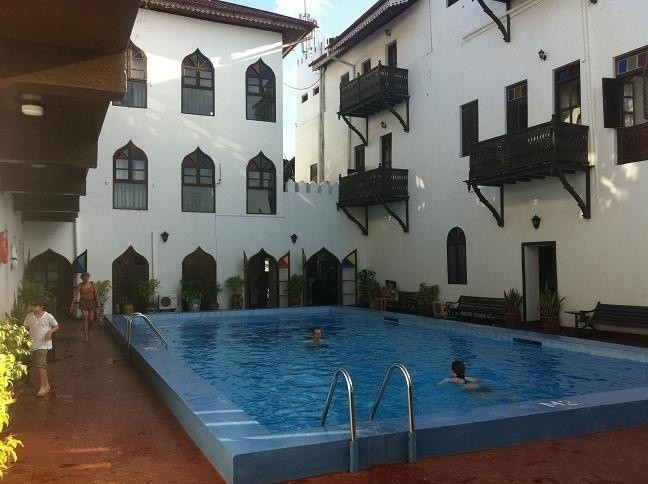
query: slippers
37 386 50 397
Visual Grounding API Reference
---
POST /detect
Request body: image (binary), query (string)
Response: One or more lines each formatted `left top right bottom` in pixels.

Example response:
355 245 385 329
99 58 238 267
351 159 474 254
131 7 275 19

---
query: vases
123 302 134 314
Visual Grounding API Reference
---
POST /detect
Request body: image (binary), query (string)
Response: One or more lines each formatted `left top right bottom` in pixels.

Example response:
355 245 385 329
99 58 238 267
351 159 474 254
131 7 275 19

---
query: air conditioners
432 301 448 319
158 293 178 310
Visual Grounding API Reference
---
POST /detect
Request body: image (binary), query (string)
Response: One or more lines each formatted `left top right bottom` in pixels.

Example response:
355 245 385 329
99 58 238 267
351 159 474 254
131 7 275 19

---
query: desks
564 311 582 328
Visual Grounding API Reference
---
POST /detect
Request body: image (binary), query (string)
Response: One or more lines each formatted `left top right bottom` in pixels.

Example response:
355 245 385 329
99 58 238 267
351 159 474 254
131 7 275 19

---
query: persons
69 272 101 342
440 360 517 403
376 280 389 309
303 327 325 345
23 296 60 397
381 281 399 311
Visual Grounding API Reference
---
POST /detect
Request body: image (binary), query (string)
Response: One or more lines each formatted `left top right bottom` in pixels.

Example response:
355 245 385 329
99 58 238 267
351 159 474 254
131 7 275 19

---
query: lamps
161 232 169 241
538 49 547 60
380 120 386 128
291 233 297 243
20 93 45 117
530 214 541 229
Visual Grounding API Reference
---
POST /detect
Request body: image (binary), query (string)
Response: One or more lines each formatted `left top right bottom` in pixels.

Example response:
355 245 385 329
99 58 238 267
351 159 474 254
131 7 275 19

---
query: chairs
373 279 397 310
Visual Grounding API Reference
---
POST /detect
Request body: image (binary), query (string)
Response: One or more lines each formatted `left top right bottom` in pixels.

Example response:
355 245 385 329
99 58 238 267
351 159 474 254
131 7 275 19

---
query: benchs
443 295 507 326
579 301 648 334
395 291 427 314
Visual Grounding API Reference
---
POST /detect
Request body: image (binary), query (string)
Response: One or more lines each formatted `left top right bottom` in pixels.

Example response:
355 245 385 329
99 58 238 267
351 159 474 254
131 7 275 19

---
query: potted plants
536 282 566 331
181 281 202 312
93 280 112 319
0 319 34 440
134 279 161 314
287 273 304 305
502 288 524 328
223 276 244 309
